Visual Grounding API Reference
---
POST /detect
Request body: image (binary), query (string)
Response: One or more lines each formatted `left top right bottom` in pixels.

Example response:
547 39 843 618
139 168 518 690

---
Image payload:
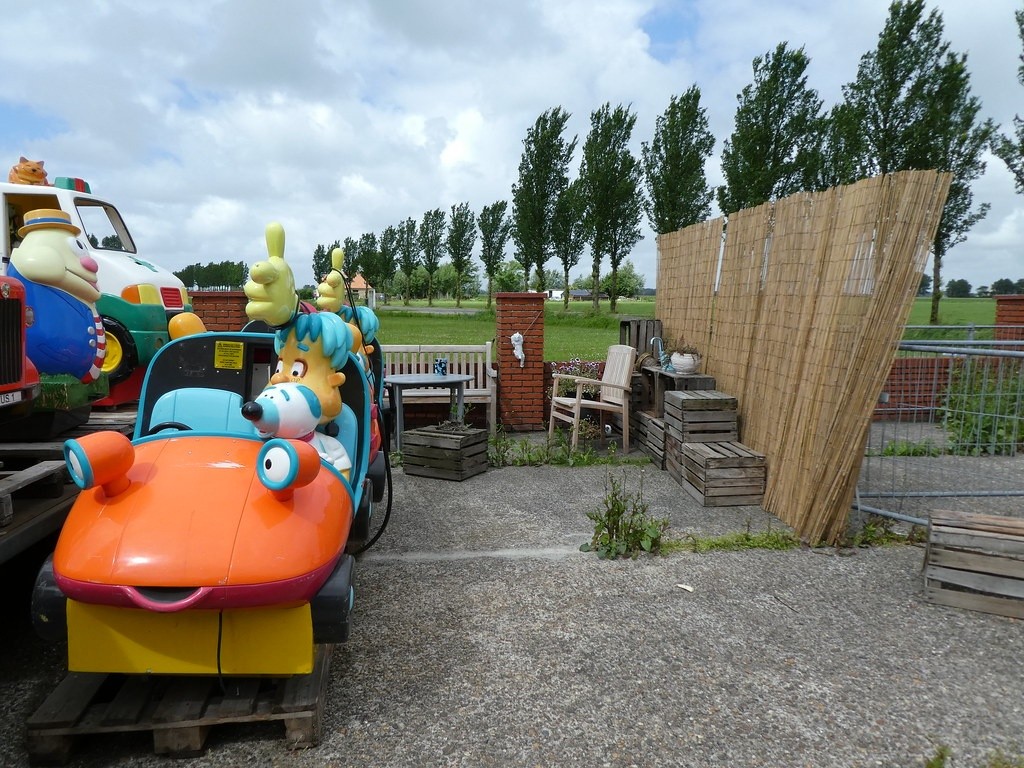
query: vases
563 392 596 432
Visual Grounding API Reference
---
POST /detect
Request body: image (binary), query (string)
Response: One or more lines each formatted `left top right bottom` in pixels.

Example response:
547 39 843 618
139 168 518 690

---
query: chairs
549 344 636 454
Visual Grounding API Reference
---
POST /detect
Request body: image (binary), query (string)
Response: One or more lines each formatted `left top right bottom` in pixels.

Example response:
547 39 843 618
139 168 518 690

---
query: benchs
149 388 357 484
380 341 498 439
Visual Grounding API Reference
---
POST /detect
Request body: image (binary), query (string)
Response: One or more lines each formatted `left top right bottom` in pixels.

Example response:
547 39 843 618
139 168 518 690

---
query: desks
384 374 475 450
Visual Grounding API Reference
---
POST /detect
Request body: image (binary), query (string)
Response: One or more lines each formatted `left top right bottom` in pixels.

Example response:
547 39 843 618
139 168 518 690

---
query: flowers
549 358 602 397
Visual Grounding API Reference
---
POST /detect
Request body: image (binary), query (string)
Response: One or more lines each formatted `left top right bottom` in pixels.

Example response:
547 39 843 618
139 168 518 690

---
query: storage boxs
612 375 765 506
918 509 1024 619
402 425 488 481
